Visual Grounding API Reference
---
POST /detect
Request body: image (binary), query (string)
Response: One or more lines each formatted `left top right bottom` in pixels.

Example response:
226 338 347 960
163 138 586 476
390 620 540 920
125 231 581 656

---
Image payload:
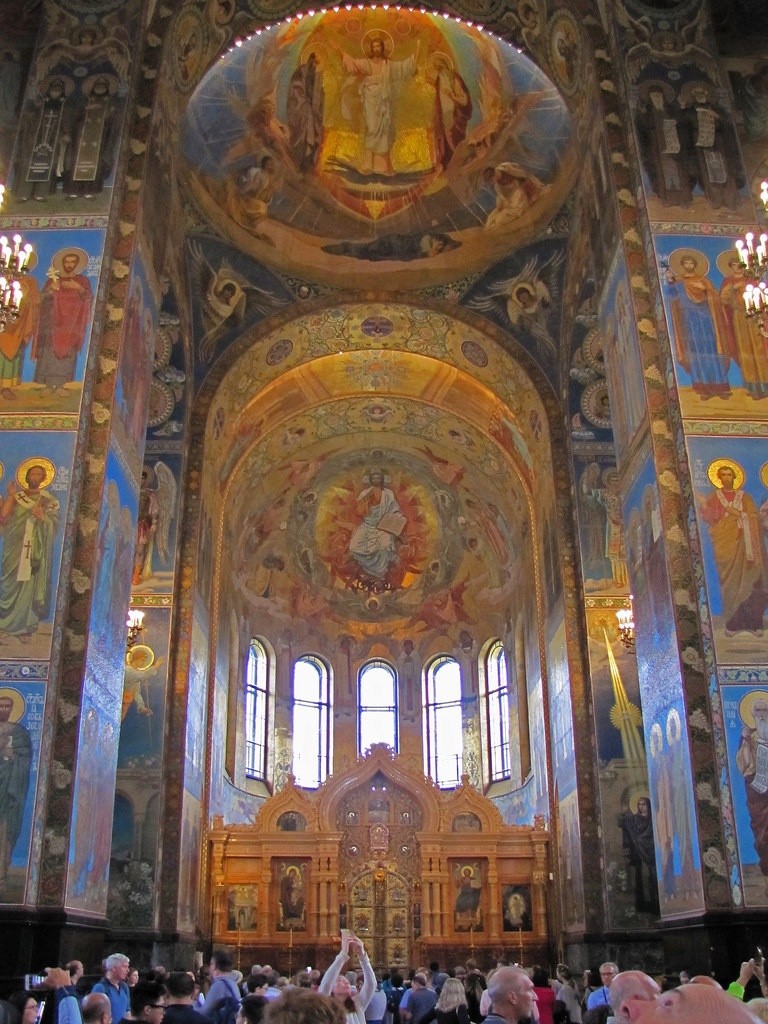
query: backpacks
212 979 242 1023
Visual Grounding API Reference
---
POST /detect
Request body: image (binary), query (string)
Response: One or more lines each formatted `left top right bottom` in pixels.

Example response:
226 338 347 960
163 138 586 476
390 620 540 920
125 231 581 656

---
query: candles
238 928 240 946
288 927 293 947
470 927 475 948
518 928 523 947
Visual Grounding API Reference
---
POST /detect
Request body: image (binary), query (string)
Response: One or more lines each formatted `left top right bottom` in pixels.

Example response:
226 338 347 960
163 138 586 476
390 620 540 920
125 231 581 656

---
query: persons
0 929 768 1024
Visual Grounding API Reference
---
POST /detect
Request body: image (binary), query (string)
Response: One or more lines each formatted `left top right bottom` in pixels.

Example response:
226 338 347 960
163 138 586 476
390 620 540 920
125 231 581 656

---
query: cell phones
34 998 46 1024
340 929 357 943
584 970 591 976
25 973 49 991
752 945 763 969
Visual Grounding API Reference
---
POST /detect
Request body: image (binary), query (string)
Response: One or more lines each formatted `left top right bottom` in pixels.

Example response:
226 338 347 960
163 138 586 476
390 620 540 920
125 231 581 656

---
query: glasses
600 972 616 978
144 1002 167 1013
25 1003 39 1010
262 987 268 992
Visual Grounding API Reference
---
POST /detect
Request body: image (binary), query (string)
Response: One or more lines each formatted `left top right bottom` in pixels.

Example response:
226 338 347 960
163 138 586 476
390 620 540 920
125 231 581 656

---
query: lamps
617 610 636 655
126 610 146 648
1 183 33 334
736 181 768 345
